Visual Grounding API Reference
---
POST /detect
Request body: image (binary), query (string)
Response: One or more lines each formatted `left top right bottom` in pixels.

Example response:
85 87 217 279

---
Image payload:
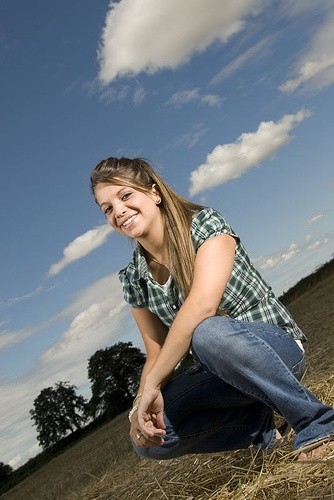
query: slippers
268 423 293 455
297 436 334 463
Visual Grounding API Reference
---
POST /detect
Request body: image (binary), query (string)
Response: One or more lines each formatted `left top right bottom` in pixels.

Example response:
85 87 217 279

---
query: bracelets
128 393 142 423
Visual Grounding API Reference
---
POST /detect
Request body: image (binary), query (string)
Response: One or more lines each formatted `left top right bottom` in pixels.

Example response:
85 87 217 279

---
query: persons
89 156 334 456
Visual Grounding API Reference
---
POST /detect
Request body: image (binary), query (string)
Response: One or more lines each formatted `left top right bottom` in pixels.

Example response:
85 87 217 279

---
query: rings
135 432 142 440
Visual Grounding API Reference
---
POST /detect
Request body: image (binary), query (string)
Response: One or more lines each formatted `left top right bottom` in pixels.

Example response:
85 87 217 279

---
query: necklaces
144 250 170 269
